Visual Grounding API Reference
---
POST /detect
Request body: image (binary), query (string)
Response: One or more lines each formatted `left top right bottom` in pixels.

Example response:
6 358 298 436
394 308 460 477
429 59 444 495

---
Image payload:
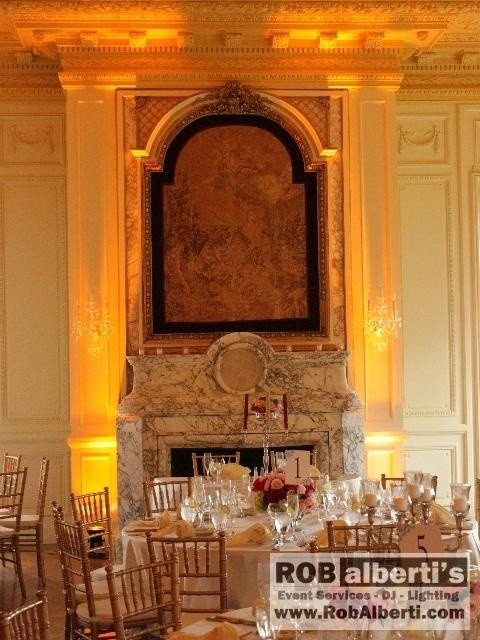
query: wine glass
179 452 479 640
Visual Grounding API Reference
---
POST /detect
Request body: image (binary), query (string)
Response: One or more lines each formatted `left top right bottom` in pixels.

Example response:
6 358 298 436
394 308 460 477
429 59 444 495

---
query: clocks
266 391 270 431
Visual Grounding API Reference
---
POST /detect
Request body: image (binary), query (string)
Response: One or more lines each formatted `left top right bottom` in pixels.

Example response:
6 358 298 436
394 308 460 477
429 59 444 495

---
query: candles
283 393 288 430
244 393 248 430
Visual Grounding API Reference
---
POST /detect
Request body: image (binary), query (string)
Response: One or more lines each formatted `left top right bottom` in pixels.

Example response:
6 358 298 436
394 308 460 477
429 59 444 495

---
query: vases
256 411 276 424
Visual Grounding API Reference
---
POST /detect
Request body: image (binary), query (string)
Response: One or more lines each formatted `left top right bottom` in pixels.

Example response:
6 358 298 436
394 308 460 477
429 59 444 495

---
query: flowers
251 397 278 416
